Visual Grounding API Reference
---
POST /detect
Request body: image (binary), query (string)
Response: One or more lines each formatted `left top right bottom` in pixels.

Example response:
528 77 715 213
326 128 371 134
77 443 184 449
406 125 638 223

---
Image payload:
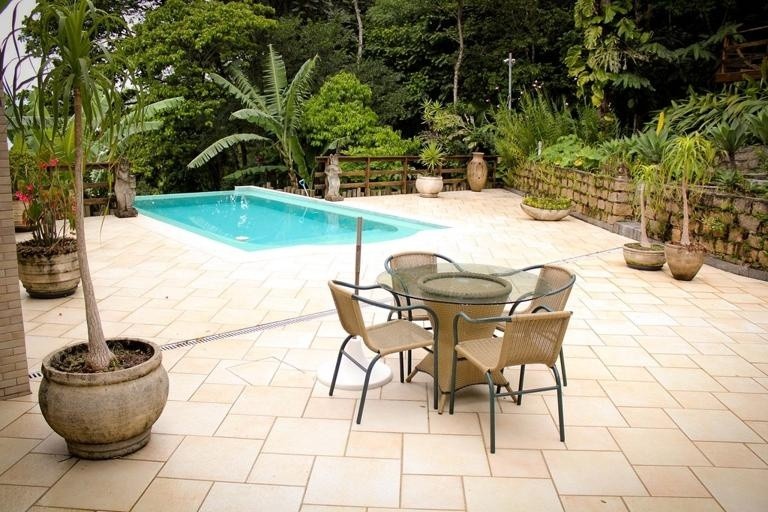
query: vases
16 239 81 298
467 152 488 192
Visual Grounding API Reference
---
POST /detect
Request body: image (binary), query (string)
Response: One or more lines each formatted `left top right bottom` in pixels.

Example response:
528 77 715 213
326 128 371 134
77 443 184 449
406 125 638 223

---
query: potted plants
0 0 169 459
662 131 710 281
415 141 446 197
623 164 666 270
520 194 571 221
9 150 37 232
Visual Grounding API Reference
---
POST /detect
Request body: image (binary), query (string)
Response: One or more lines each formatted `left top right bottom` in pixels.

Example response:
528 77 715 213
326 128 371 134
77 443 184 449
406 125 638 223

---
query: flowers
16 159 79 246
